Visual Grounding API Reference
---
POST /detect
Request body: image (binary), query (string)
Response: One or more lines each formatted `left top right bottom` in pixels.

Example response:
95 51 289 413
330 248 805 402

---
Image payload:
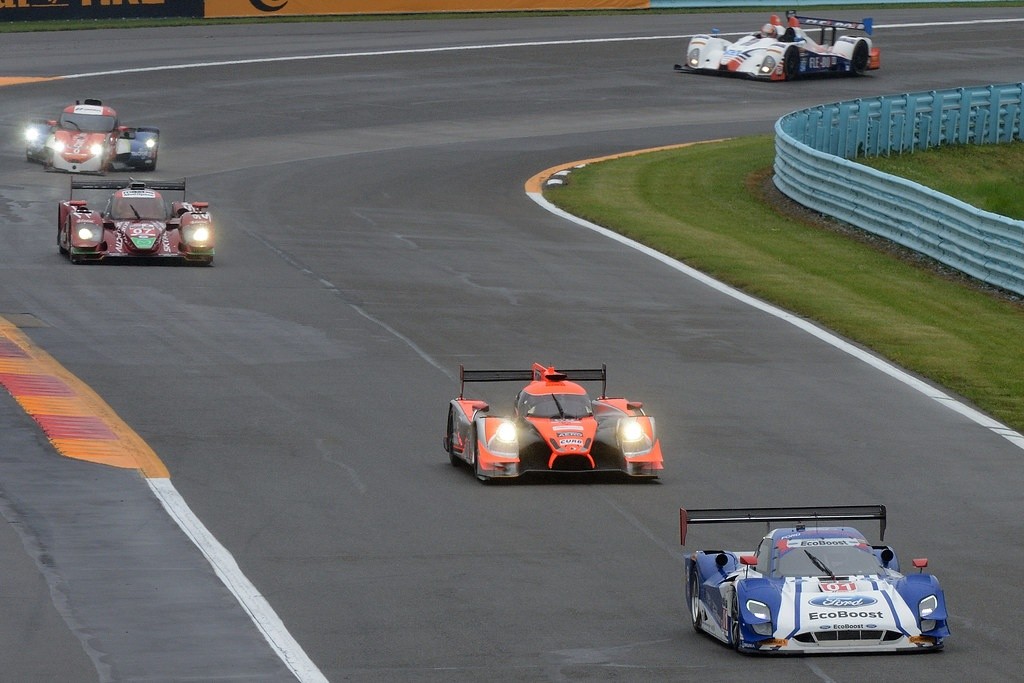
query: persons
117 200 136 215
760 23 781 39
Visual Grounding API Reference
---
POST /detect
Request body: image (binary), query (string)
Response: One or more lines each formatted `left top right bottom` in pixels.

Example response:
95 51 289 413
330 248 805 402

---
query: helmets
761 23 778 39
824 547 846 568
